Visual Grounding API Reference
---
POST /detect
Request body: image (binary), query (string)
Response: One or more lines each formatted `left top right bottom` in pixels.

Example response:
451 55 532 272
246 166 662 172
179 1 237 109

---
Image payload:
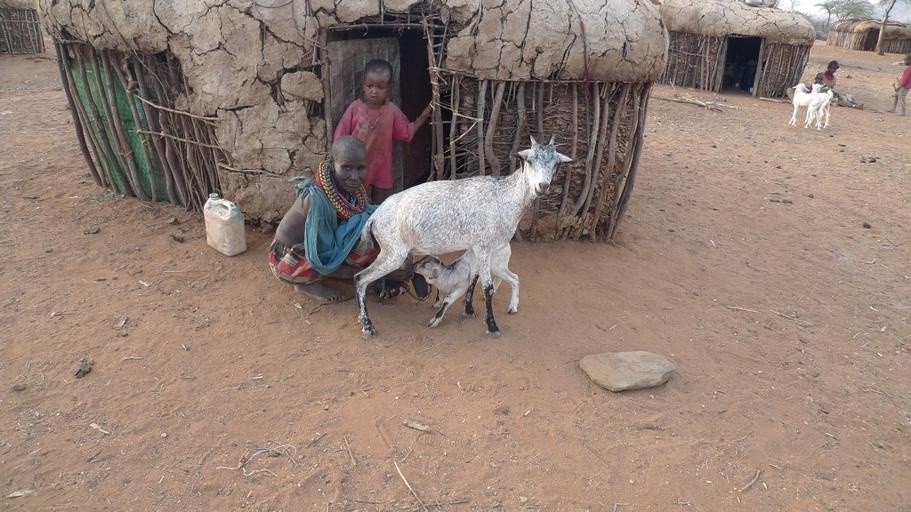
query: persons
887 53 911 116
268 135 414 302
806 61 863 111
333 59 433 205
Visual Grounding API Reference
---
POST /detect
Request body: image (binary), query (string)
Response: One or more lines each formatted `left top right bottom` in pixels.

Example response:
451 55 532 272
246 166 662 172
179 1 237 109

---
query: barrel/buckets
203 192 248 256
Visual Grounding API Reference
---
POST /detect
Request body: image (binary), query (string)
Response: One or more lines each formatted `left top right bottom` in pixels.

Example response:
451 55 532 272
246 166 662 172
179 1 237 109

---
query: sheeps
352 132 576 337
788 82 834 131
411 240 520 328
